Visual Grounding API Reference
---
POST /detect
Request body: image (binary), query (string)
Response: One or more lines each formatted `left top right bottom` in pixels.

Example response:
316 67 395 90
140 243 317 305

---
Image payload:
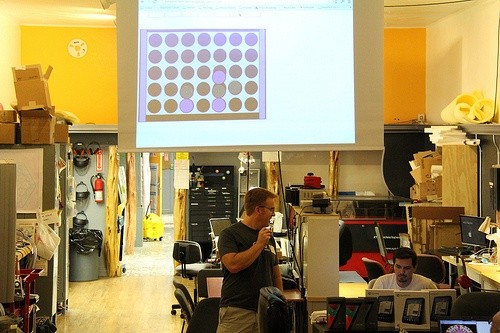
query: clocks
68 39 88 59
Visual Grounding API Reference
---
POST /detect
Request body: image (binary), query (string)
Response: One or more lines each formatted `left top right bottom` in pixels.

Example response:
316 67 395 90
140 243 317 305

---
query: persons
216 188 283 333
373 247 438 291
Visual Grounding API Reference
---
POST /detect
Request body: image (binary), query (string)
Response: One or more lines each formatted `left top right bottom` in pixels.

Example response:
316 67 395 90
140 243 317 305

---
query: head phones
72 141 100 155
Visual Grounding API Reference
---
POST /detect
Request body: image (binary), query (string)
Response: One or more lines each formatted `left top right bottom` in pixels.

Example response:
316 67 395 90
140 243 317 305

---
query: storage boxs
394 291 431 330
365 289 395 328
408 149 442 205
0 63 69 145
421 288 456 328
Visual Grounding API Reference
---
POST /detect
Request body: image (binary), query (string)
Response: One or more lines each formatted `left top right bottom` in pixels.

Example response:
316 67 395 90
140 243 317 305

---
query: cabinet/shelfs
411 145 478 255
189 165 234 263
288 204 341 333
10 268 45 333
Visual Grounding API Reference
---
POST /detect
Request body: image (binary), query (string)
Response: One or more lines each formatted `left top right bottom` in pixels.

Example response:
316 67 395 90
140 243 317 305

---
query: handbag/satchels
31 212 61 261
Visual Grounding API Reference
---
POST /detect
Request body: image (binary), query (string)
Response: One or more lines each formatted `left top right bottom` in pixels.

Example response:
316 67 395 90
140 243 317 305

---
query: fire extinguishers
94 173 104 203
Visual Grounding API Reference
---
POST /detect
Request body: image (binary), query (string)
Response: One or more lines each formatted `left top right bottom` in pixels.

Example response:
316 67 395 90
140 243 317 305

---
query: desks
339 280 370 301
480 269 500 292
206 276 304 333
442 256 480 288
465 263 500 285
342 220 407 225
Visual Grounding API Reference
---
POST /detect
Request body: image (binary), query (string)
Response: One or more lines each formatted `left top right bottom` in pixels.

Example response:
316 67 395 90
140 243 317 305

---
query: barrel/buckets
76 182 89 198
74 149 90 168
73 211 88 226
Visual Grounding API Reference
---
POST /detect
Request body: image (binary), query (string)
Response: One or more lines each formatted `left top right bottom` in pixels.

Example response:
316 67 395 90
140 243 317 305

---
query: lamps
478 216 498 234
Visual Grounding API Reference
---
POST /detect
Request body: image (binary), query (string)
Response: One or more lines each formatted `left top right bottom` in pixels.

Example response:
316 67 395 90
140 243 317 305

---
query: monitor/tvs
209 218 232 251
459 214 489 253
376 295 395 323
402 298 425 324
430 296 452 322
365 288 492 333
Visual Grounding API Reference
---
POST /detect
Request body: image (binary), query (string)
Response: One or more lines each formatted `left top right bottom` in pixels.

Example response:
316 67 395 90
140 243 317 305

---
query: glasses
253 205 275 214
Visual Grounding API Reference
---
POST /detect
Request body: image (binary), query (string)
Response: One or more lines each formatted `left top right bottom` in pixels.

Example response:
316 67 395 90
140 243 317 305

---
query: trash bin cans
69 228 101 281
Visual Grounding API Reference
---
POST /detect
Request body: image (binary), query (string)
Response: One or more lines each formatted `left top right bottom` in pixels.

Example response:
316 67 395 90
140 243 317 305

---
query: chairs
375 227 402 266
174 288 193 333
451 292 500 322
171 279 194 316
187 297 220 333
361 257 385 279
414 254 445 283
